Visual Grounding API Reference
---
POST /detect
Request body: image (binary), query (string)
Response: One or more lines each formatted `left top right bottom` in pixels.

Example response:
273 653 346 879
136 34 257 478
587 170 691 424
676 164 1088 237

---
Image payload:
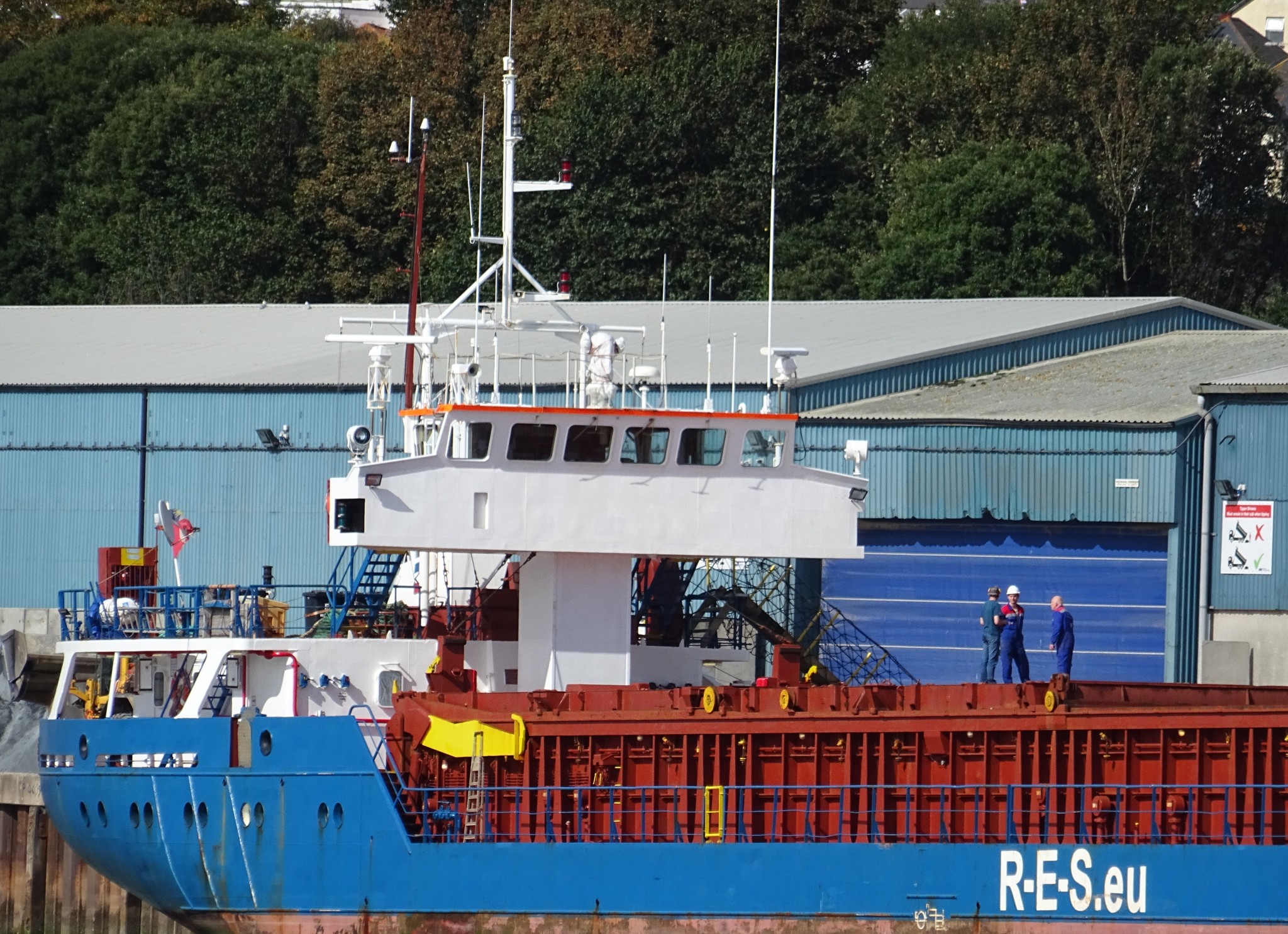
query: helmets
1006 585 1020 595
988 586 1001 596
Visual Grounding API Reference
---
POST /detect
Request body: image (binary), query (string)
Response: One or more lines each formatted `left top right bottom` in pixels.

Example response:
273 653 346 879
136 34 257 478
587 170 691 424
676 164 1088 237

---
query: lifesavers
170 668 191 710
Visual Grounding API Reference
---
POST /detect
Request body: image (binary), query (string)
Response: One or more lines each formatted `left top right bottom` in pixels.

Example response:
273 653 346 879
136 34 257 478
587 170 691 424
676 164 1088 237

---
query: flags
172 508 194 558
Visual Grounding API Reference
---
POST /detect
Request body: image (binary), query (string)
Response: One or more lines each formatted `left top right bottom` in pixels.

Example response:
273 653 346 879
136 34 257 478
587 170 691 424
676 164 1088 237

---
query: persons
980 586 1007 683
1000 585 1031 684
1049 595 1075 680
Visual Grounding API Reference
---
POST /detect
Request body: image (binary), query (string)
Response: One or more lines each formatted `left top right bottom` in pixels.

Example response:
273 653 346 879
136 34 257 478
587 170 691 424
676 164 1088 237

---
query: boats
0 0 1288 934
